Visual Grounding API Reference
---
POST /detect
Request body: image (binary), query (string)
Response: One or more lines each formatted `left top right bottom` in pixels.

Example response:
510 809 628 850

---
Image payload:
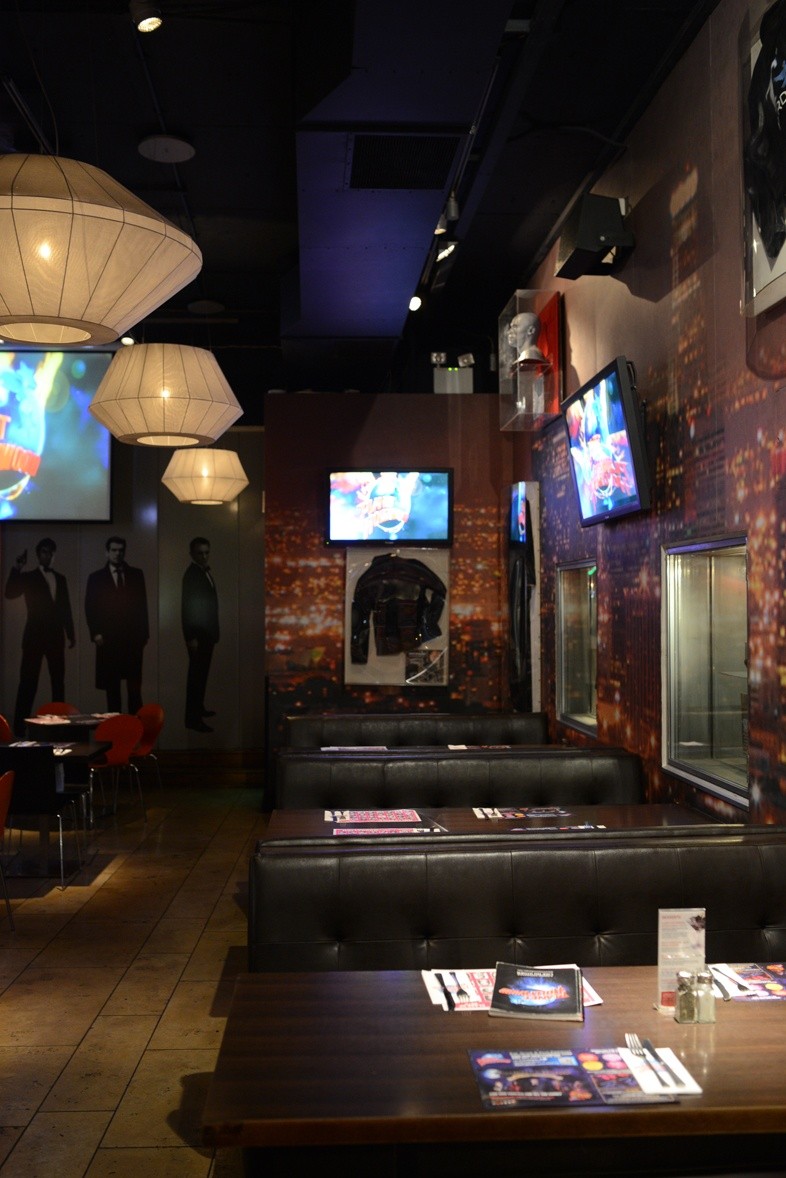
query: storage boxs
499 287 561 433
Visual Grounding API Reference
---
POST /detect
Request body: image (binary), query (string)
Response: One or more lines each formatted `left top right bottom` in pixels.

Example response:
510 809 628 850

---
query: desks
199 963 785 1152
264 799 725 840
327 741 576 751
24 715 106 793
0 741 111 890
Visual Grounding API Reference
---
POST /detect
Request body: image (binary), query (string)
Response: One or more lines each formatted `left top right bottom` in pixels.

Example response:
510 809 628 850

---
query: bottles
695 972 716 1024
674 971 697 1023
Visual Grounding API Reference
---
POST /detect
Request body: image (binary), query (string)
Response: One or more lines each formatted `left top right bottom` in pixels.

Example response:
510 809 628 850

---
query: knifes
704 965 731 1000
434 973 456 1012
643 1039 684 1085
331 810 337 823
480 808 488 820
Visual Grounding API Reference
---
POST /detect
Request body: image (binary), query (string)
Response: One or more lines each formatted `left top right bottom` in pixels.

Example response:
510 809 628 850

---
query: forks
449 972 470 1002
712 967 751 991
491 807 497 816
624 1033 669 1087
340 810 346 820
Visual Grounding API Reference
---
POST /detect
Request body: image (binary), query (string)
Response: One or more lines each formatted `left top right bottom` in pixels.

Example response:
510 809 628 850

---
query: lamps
0 158 202 349
162 451 250 506
434 213 449 235
446 193 462 222
430 350 476 396
88 338 245 448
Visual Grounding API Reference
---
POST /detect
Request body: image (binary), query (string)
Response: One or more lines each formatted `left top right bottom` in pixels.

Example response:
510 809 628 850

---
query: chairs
0 703 165 857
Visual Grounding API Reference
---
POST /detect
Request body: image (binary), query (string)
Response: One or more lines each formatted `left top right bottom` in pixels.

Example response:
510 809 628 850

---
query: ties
117 569 123 592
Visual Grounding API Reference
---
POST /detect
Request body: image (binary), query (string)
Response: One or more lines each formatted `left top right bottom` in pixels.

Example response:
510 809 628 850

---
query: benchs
249 824 785 975
276 744 642 808
287 711 557 753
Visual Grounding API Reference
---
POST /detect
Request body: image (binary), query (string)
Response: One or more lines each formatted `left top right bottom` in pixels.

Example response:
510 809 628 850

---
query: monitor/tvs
560 354 654 528
321 466 454 548
0 346 117 526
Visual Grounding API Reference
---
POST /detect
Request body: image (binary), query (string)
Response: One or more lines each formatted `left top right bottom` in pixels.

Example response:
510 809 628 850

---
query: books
489 961 583 1021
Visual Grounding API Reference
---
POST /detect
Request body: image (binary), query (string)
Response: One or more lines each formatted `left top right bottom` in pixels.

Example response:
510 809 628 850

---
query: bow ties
203 566 210 572
44 567 53 571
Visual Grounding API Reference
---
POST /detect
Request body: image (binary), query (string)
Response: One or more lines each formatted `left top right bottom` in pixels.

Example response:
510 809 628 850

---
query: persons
181 537 220 733
4 538 75 738
84 536 151 746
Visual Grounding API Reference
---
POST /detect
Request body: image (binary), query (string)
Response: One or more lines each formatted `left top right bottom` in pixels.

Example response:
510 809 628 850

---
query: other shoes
200 709 215 716
185 716 213 733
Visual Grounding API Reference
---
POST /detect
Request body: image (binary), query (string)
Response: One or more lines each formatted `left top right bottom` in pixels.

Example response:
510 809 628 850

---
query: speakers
553 192 635 280
